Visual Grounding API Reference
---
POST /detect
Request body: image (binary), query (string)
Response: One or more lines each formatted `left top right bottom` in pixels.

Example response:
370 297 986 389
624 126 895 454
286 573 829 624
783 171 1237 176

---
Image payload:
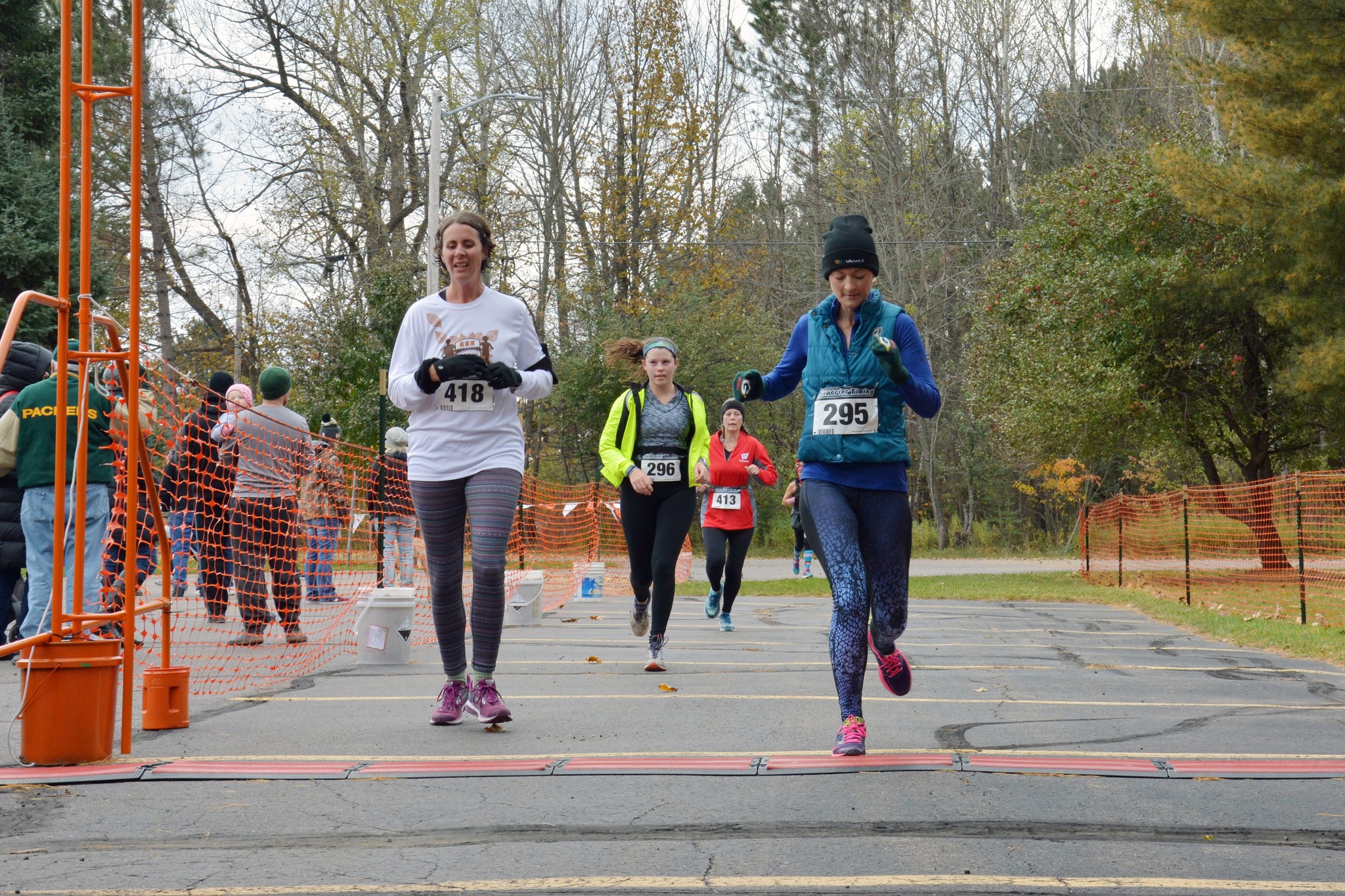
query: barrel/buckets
15 638 121 765
572 562 605 602
675 553 692 582
503 570 543 625
354 586 416 665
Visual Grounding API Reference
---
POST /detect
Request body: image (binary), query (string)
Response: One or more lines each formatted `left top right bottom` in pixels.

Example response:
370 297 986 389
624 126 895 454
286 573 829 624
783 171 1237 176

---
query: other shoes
227 627 264 645
285 629 307 643
155 574 350 623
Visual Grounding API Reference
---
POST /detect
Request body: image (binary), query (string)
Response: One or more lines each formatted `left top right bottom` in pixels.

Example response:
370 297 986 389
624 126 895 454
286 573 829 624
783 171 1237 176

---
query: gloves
480 361 521 390
221 421 234 437
138 364 149 387
733 370 766 402
869 336 908 386
433 354 485 383
786 493 800 505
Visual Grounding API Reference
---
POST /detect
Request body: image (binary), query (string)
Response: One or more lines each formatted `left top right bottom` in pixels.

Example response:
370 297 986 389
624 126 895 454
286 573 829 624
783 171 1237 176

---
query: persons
732 214 942 757
597 336 711 674
218 365 308 646
159 372 252 625
300 414 351 603
778 455 817 579
0 338 158 664
696 398 778 631
390 211 558 726
364 424 417 589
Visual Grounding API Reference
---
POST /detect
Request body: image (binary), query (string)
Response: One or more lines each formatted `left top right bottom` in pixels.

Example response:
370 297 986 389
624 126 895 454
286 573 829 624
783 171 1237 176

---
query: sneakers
867 628 912 698
719 613 736 631
802 568 813 579
792 556 800 575
832 714 868 757
705 582 724 618
644 633 668 671
430 672 472 725
630 591 651 637
465 678 512 723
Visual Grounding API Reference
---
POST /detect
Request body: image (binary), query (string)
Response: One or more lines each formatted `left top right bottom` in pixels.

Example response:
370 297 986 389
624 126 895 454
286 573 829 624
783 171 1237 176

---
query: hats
821 215 879 281
319 414 343 440
385 426 408 450
52 338 80 371
794 459 803 472
209 365 292 413
103 363 120 385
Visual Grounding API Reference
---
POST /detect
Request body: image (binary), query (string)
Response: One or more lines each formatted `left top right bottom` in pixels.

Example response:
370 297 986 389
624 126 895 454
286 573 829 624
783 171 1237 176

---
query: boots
98 569 144 647
